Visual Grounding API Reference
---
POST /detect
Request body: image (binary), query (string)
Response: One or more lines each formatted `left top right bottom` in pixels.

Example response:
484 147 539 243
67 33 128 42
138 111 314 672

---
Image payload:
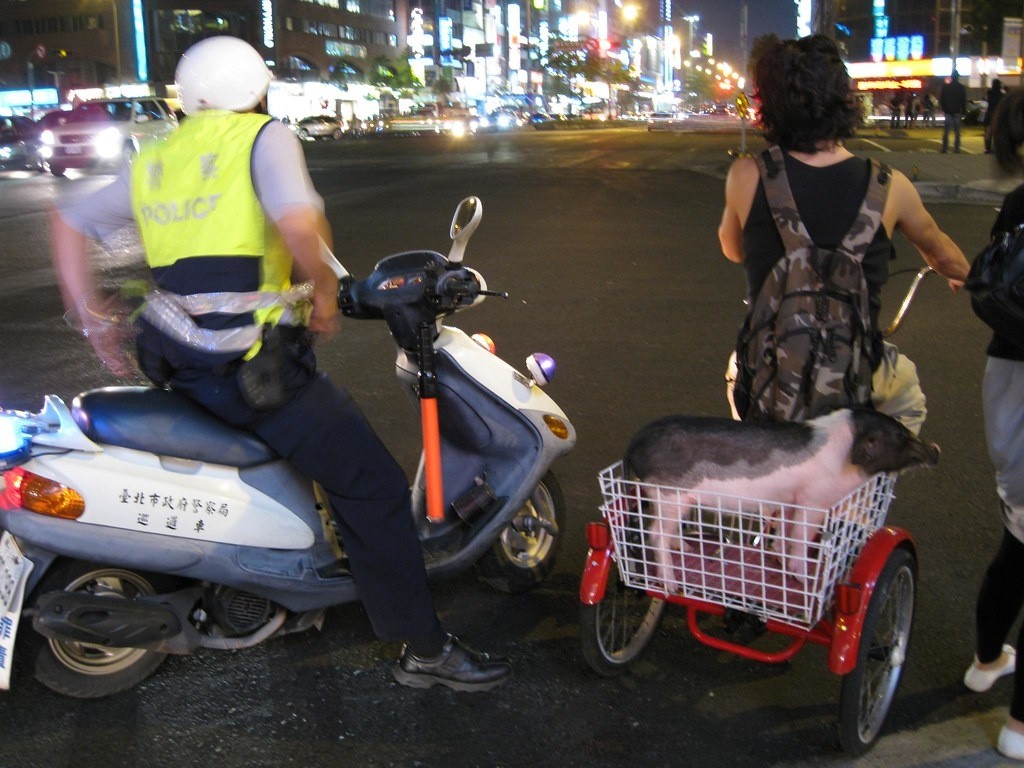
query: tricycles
580 264 943 755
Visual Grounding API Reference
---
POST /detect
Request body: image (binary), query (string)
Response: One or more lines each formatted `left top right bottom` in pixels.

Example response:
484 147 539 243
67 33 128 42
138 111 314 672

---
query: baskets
599 460 893 631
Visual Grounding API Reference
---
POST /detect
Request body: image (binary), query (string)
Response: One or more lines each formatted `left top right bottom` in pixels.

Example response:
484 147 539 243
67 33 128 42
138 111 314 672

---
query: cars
299 116 343 142
479 106 581 130
1 109 74 173
647 113 674 130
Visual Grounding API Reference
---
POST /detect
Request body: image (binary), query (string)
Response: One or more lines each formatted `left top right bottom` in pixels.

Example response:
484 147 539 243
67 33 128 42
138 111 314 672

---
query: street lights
527 0 546 93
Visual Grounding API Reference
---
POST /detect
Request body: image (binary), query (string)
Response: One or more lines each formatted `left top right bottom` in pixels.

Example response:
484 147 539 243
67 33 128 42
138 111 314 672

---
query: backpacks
741 145 891 423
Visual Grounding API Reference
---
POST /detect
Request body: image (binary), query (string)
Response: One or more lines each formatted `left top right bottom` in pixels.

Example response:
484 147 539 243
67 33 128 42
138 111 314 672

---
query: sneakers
389 643 512 691
997 725 1024 761
965 644 1019 692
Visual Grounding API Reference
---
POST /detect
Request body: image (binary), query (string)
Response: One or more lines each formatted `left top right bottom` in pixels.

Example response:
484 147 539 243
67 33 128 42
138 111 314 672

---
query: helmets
178 36 271 114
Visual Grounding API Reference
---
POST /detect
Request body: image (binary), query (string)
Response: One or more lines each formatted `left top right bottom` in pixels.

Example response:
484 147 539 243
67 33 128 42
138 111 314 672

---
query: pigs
621 385 942 596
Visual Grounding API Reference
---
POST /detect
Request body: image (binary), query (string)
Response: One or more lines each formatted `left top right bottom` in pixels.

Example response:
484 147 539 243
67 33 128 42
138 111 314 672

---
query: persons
47 35 514 693
344 111 416 139
983 80 1007 155
281 117 300 125
887 89 939 129
718 34 972 435
964 87 1024 763
938 69 966 154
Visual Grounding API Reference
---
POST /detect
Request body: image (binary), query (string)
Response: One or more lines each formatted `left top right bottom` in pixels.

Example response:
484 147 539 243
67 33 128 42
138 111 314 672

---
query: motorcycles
0 194 575 706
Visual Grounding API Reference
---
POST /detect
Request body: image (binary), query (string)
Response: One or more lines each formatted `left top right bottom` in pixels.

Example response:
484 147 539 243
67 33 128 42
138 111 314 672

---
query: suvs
39 96 180 178
